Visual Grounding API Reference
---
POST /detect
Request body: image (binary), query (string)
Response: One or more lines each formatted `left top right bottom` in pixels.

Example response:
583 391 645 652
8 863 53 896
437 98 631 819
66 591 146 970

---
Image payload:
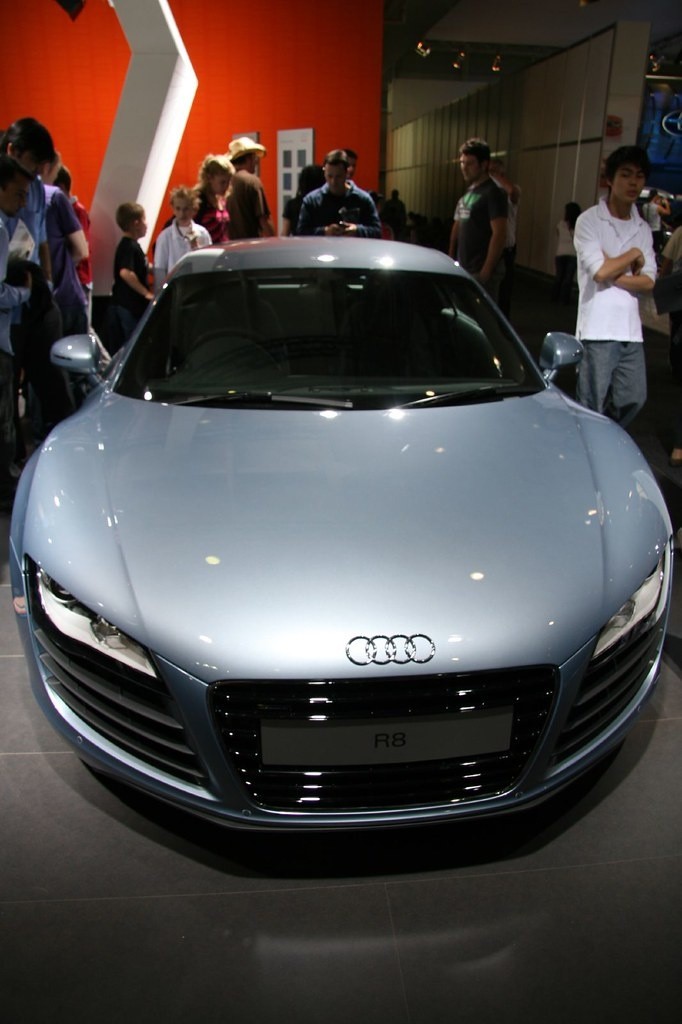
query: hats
224 137 266 162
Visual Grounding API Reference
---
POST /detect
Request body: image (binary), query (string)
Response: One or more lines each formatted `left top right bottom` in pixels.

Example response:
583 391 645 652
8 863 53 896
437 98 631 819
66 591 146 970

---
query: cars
10 234 679 834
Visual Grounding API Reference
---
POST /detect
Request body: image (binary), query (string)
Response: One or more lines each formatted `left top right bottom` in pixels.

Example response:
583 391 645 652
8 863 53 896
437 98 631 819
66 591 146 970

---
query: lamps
412 41 666 73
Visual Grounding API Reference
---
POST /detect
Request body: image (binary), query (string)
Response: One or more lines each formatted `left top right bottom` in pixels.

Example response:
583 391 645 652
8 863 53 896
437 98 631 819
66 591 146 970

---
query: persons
449 137 521 320
283 149 407 239
555 145 682 467
111 136 274 342
0 118 111 511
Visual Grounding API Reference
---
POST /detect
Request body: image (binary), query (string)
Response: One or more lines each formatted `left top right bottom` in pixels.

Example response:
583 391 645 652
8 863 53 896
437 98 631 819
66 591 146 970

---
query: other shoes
670 447 682 467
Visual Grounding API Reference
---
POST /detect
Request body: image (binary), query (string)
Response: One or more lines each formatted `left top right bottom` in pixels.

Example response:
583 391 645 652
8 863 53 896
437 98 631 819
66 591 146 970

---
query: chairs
169 274 498 391
